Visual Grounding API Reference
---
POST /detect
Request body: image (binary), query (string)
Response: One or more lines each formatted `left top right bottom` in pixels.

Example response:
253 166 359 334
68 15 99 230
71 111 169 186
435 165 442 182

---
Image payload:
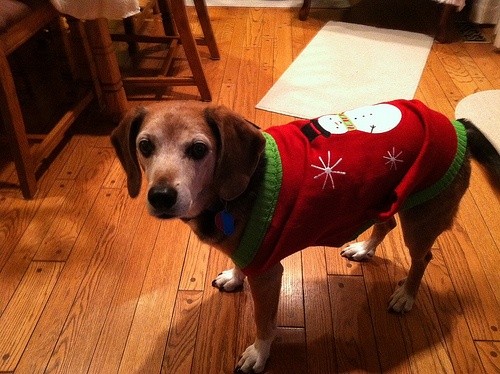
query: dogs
110 99 500 373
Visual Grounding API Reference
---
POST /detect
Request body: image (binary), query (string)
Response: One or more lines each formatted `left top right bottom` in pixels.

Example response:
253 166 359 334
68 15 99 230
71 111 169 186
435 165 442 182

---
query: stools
299 0 454 44
0 0 221 200
